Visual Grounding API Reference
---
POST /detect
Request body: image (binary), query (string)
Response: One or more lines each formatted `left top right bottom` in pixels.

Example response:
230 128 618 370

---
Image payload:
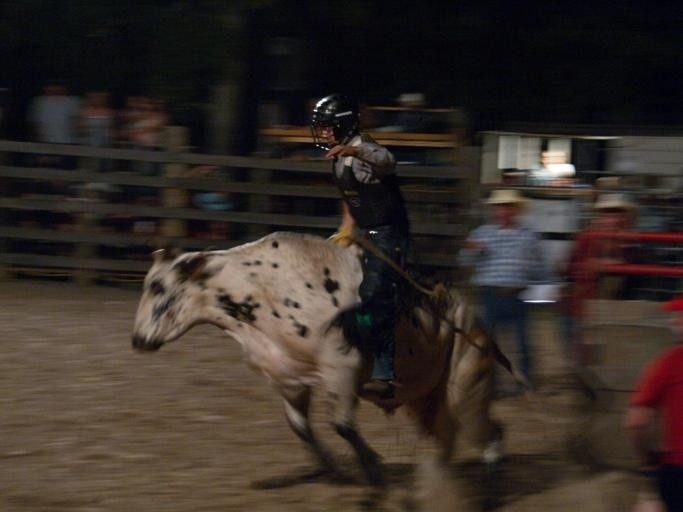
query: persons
552 190 640 468
446 186 549 391
615 294 682 511
305 93 416 396
0 61 683 302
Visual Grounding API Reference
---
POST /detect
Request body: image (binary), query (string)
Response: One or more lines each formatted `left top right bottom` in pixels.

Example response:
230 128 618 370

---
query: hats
484 188 528 208
660 294 683 312
584 192 636 215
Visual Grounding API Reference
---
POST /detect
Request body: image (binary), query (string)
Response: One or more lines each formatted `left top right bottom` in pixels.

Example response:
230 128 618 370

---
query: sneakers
362 380 396 398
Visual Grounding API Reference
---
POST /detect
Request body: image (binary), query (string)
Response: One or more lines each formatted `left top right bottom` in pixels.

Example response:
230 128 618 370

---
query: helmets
310 94 362 143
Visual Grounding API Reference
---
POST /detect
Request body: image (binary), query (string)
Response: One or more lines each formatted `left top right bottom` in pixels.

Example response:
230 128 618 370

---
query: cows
126 230 532 486
